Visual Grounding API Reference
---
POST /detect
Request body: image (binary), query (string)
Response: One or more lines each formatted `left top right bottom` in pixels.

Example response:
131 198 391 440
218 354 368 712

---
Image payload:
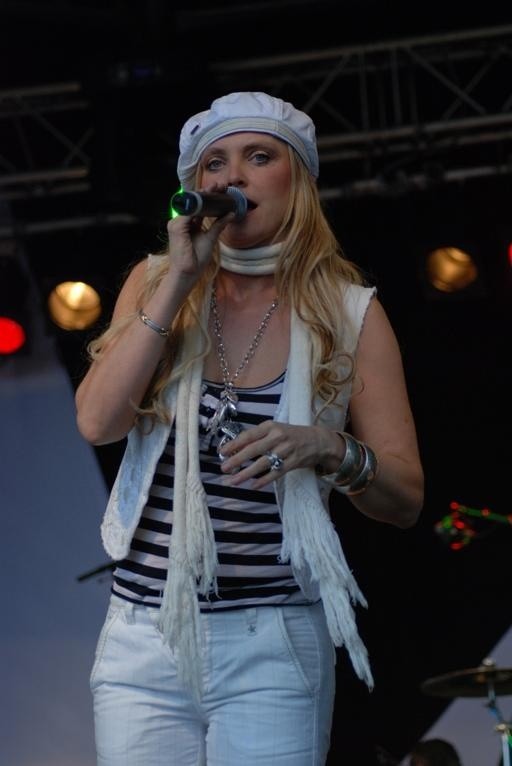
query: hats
177 91 320 191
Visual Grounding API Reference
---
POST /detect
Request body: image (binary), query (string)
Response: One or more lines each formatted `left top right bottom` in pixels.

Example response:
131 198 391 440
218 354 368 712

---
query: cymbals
421 665 511 700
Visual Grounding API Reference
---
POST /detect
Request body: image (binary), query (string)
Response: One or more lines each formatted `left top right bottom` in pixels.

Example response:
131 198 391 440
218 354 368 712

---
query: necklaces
204 282 279 476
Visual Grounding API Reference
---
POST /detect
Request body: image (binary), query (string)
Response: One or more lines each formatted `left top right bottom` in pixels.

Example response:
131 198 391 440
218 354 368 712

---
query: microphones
171 186 252 223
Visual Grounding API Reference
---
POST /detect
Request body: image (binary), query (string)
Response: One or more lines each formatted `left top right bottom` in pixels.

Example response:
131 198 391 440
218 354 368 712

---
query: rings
267 454 284 469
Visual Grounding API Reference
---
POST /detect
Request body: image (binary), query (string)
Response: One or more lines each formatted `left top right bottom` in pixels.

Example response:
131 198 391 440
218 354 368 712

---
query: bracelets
138 307 170 340
314 430 360 487
334 440 378 496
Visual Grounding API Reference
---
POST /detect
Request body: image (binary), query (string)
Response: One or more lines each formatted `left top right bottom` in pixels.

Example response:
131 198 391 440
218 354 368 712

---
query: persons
73 92 426 766
